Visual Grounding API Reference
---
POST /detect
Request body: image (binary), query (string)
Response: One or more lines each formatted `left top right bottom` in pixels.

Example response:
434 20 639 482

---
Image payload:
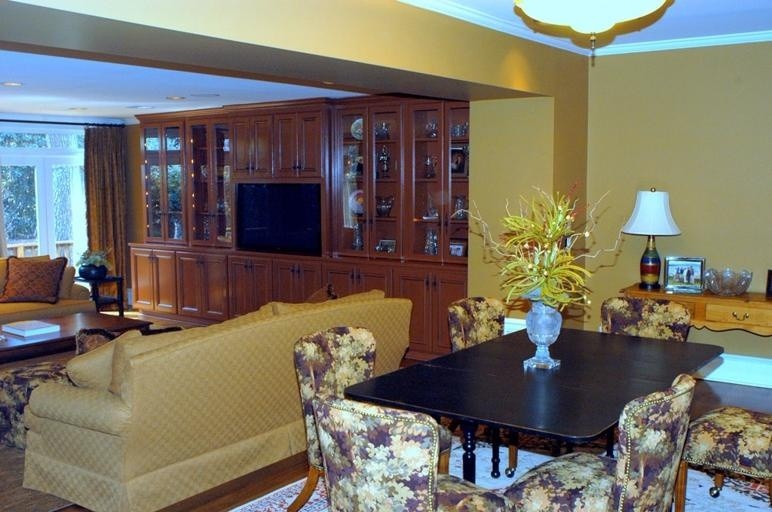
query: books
2 320 61 338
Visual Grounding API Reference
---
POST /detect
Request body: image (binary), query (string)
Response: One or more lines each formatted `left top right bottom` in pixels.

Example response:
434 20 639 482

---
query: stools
0 362 77 450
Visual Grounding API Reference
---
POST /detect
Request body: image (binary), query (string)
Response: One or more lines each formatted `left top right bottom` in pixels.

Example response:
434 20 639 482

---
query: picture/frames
664 257 706 291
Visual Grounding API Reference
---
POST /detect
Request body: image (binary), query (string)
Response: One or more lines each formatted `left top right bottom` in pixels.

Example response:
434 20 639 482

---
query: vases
520 287 563 369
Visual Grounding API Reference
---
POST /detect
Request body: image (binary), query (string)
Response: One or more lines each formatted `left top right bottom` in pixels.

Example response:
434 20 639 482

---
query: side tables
74 277 124 318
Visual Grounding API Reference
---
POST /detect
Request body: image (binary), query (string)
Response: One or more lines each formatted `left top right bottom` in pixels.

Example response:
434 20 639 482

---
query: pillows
65 304 273 391
271 289 386 317
0 254 76 304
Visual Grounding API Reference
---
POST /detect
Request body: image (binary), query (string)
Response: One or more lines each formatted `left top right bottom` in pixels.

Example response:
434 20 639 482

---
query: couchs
0 255 96 323
21 289 413 512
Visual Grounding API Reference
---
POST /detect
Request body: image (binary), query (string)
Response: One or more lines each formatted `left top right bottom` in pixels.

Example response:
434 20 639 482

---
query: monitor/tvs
234 183 322 257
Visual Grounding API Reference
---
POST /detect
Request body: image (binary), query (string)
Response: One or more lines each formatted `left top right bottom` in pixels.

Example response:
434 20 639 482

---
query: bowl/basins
705 268 753 298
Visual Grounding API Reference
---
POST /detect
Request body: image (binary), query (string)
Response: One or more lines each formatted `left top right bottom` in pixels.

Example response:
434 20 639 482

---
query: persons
673 265 695 285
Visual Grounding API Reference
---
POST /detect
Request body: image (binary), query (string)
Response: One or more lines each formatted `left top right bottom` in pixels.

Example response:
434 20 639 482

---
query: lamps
512 0 676 50
620 188 682 290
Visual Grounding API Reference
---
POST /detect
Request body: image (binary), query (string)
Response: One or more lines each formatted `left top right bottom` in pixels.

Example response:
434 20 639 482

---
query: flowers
449 182 626 311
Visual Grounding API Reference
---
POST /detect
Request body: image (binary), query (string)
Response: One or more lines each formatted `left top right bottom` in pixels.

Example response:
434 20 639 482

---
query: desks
619 282 772 337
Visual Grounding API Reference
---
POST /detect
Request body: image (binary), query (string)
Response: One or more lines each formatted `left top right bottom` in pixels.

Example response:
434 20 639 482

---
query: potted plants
75 248 112 281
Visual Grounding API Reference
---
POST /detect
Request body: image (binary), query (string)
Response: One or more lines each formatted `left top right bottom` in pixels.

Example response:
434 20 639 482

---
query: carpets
0 312 209 512
231 436 772 512
